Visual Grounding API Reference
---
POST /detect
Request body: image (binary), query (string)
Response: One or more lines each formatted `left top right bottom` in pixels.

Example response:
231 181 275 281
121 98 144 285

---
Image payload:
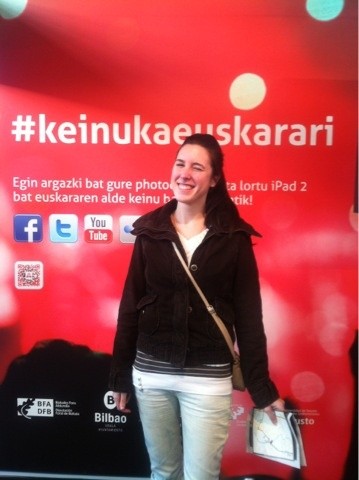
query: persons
107 133 288 480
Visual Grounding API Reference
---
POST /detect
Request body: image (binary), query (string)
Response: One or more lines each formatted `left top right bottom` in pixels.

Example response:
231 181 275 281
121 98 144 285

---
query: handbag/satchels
233 351 245 391
